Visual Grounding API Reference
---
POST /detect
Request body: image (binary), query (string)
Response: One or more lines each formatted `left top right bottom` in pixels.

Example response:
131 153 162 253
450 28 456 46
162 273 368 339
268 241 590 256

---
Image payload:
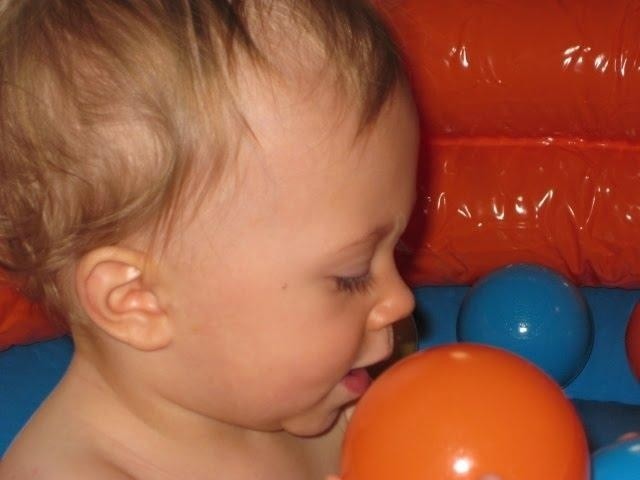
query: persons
0 0 421 478
